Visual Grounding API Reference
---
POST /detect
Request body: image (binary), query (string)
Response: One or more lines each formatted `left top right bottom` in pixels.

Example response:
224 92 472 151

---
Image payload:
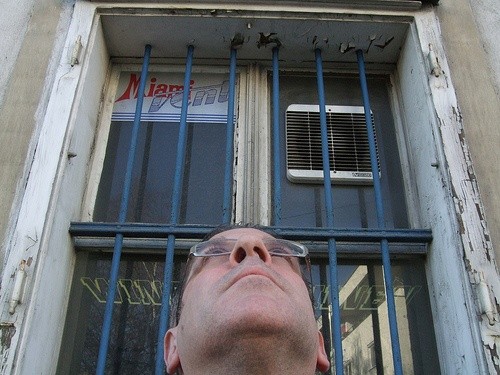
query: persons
163 220 330 375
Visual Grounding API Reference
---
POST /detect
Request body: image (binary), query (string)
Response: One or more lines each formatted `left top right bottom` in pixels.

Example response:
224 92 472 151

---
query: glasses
174 238 316 323
